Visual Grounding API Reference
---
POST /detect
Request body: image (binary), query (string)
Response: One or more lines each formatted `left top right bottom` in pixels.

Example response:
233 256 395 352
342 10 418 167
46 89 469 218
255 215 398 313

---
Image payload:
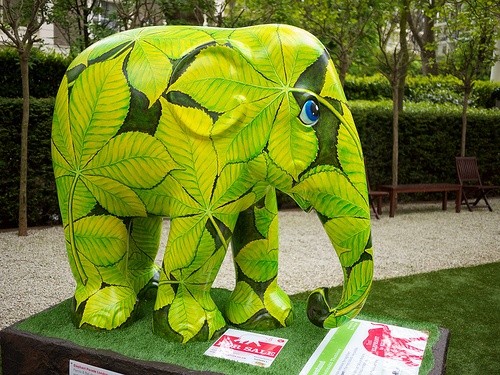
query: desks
378 183 464 218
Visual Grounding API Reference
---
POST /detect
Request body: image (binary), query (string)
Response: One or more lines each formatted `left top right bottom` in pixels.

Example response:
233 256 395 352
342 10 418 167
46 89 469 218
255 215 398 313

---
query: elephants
51 26 374 345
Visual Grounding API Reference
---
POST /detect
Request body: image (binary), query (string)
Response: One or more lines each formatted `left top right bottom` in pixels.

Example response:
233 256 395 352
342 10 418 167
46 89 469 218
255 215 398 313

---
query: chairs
456 157 500 212
364 156 389 219
